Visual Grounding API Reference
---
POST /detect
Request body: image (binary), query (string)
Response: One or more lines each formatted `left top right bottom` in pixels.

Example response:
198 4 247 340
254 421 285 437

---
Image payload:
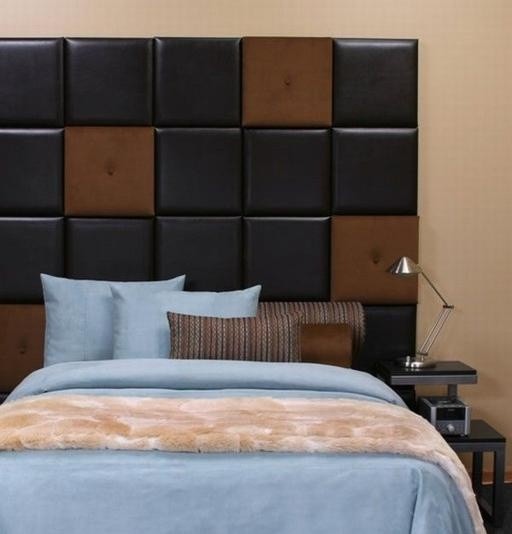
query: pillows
40 271 188 362
109 283 262 358
166 312 303 363
303 323 353 366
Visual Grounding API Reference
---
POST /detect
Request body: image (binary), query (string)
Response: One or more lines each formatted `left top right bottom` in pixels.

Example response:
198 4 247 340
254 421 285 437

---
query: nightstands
386 360 506 534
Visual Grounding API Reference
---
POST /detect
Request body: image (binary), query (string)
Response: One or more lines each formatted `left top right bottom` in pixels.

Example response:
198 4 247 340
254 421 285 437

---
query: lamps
387 256 454 367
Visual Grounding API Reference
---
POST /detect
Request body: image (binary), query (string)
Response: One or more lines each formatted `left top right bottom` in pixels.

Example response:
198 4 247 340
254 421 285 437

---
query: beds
0 37 486 534
